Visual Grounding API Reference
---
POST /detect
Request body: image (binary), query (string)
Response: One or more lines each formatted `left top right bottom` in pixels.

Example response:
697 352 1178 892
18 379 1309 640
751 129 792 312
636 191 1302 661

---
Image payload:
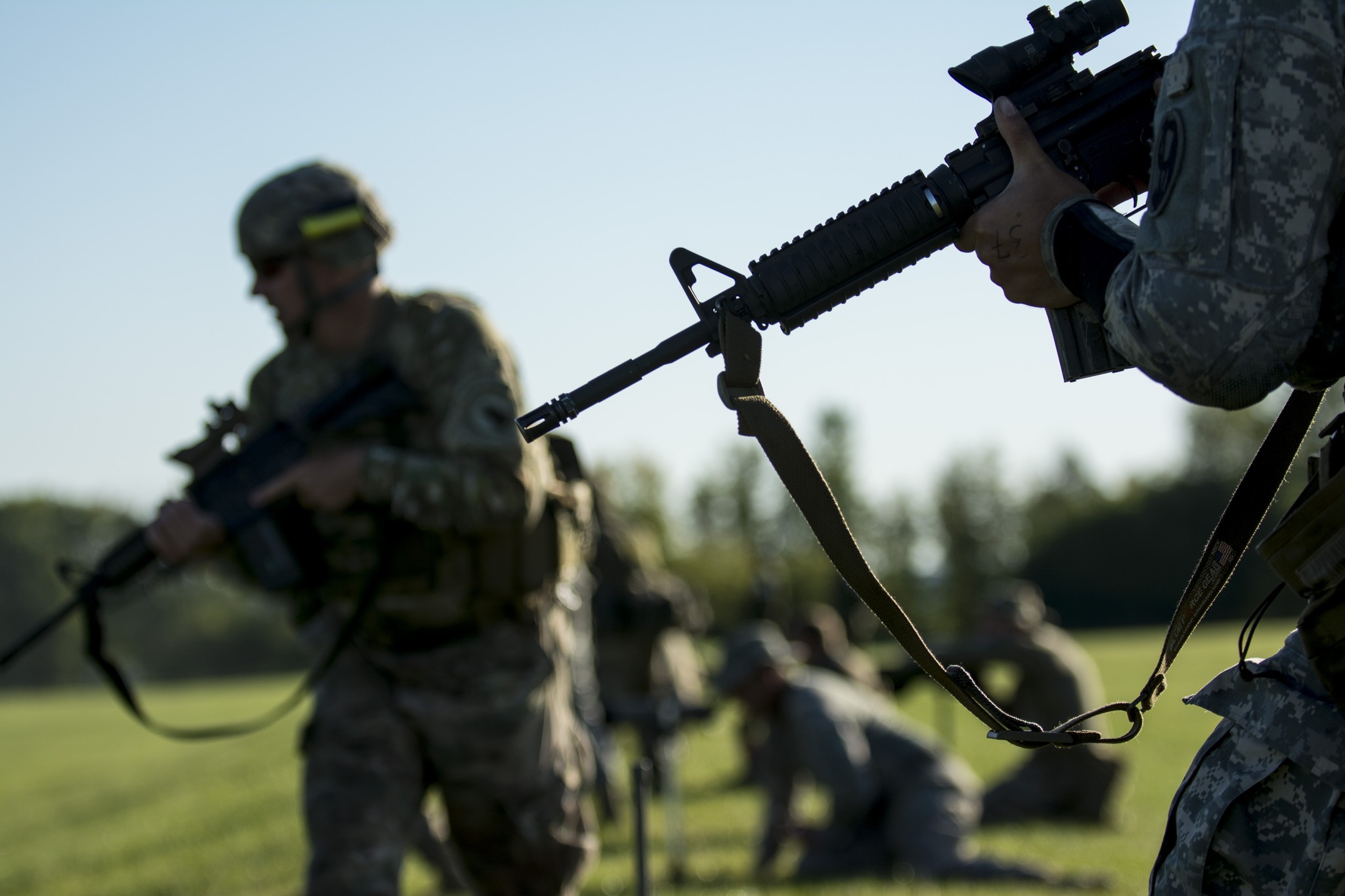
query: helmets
986 577 1045 642
724 631 791 694
236 165 393 265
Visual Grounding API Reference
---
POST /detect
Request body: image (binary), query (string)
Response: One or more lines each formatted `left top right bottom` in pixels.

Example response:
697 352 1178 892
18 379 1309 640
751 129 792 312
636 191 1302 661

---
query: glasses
250 249 294 282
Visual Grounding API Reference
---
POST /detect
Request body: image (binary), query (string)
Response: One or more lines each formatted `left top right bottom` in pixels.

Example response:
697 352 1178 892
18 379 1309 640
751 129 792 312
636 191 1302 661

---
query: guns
512 0 1188 448
0 344 433 692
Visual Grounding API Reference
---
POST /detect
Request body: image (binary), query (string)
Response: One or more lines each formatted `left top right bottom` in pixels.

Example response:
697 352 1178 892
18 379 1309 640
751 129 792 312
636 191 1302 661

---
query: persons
941 0 1345 896
549 468 625 851
589 522 719 885
718 629 1052 891
973 571 1119 834
729 600 888 794
141 157 615 896
